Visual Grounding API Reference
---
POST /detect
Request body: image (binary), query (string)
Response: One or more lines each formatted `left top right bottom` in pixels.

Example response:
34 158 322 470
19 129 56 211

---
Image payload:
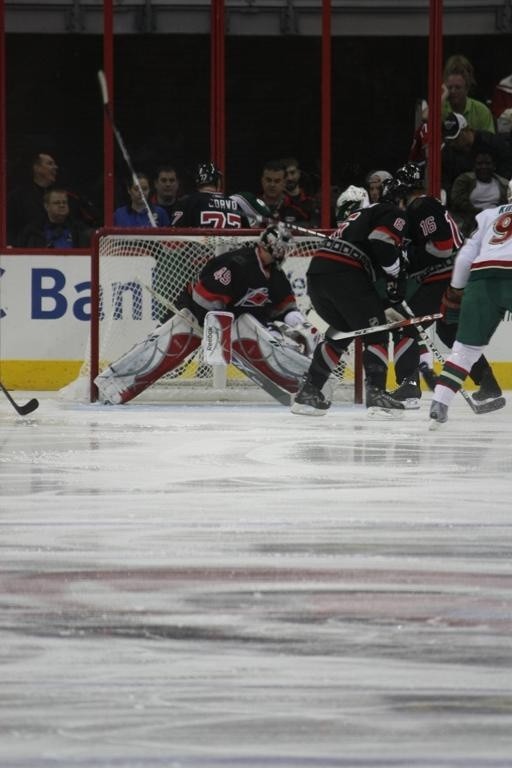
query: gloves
386 267 406 304
441 287 463 323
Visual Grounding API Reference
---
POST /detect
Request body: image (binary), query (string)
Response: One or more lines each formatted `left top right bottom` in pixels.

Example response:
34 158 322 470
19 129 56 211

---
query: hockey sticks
136 275 334 406
401 300 506 413
305 307 444 340
0 381 39 415
97 70 158 227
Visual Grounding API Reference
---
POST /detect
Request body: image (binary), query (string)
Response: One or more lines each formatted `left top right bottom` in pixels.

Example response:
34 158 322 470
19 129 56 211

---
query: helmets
195 161 424 261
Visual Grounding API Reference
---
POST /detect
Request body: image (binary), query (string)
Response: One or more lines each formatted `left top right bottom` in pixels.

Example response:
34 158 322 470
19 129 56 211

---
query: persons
10 53 511 423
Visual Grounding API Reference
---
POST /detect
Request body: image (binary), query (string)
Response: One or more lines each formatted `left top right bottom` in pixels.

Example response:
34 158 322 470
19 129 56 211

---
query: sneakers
295 367 502 423
